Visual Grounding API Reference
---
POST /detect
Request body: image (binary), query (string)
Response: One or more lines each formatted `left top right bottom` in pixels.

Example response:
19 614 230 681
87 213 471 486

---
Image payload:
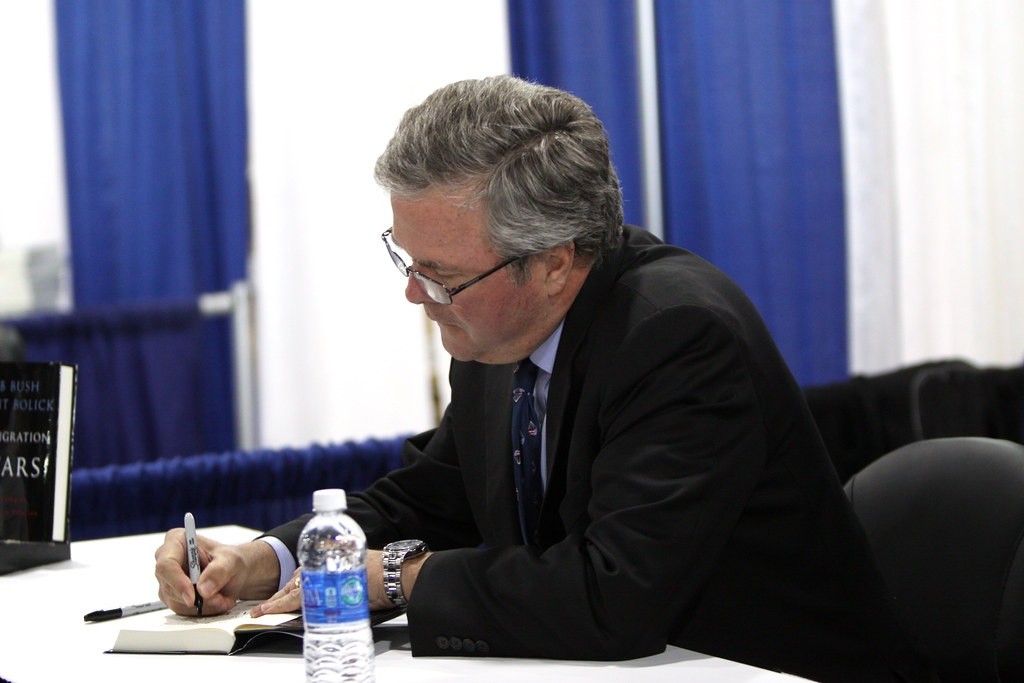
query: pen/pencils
180 511 208 617
82 597 168 625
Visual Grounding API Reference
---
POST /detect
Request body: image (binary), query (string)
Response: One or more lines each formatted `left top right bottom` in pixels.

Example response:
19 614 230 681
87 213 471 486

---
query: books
1 356 79 546
105 598 408 657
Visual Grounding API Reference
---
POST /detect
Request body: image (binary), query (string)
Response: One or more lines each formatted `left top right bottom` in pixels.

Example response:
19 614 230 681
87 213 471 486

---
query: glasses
381 227 525 305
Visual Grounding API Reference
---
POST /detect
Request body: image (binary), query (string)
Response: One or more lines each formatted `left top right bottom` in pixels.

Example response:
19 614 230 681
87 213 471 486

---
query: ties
511 357 545 550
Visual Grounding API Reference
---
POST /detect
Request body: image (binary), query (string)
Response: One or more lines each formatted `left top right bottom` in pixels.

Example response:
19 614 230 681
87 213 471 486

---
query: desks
0 521 821 683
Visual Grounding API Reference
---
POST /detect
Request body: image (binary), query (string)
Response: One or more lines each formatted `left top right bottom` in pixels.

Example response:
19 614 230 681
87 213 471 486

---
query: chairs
841 433 1024 683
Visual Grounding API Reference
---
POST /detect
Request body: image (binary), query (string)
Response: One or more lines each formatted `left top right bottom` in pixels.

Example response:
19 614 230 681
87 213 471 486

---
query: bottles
296 489 375 683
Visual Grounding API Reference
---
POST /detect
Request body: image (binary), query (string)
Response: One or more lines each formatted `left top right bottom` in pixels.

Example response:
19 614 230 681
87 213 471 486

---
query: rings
294 579 300 588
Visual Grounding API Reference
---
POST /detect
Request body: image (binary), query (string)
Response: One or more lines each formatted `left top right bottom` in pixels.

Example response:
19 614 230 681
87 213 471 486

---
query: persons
156 74 916 682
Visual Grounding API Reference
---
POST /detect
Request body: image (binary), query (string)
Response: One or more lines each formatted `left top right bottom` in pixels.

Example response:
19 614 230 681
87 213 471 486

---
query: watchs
381 535 424 608
